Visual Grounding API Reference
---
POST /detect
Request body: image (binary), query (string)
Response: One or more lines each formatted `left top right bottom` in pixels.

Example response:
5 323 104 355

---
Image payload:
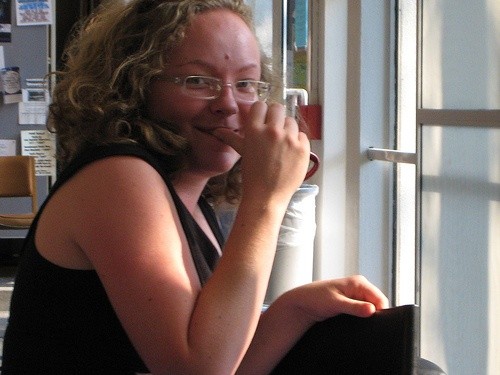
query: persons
2 0 392 374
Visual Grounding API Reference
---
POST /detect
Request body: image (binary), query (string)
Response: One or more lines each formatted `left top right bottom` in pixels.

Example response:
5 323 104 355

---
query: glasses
157 73 271 104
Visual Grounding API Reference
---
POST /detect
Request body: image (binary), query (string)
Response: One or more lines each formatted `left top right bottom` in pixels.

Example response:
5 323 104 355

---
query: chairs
2 155 38 230
